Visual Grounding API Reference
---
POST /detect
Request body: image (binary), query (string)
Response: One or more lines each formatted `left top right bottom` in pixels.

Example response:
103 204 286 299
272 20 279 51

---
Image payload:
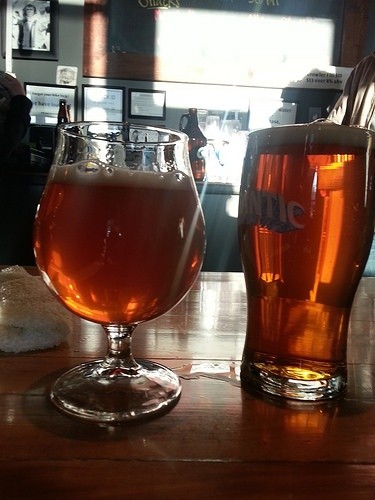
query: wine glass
34 122 206 422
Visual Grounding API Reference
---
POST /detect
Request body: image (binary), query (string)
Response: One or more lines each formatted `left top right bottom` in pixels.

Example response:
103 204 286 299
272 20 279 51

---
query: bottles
53 99 69 156
180 109 207 181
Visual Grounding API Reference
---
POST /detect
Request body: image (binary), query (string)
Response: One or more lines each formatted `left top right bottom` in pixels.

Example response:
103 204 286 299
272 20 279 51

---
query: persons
13 4 51 49
0 73 41 267
322 49 375 277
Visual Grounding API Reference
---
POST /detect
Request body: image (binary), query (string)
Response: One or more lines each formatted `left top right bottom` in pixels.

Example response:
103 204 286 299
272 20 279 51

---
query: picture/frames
23 82 79 125
82 84 127 123
128 88 167 120
3 0 59 61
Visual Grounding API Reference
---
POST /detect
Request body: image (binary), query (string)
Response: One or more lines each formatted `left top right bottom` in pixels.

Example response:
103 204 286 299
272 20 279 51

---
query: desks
0 264 375 500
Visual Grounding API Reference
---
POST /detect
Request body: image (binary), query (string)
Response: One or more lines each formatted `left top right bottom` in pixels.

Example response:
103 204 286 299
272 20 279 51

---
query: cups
238 124 375 410
